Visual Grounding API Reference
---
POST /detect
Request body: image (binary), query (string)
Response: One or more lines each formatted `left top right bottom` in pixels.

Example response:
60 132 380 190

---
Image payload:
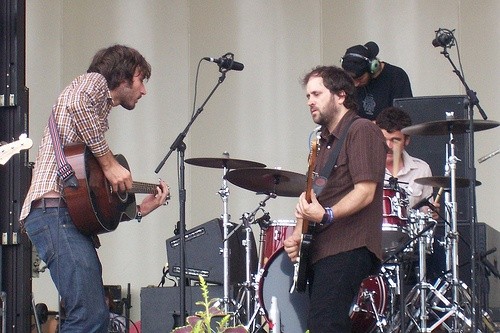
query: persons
21 45 170 333
341 42 413 122
370 107 439 325
283 67 387 333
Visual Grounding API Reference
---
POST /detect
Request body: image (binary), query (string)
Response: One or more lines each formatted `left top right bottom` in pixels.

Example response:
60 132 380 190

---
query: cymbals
225 168 308 196
413 176 483 188
401 120 500 136
184 158 266 169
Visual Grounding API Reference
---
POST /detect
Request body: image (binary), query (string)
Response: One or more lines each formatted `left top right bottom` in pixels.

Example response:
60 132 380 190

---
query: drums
262 220 298 270
405 211 436 262
256 244 388 333
381 186 408 250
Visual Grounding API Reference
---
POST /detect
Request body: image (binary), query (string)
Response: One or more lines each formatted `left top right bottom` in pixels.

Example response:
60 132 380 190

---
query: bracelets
319 207 328 227
135 205 142 222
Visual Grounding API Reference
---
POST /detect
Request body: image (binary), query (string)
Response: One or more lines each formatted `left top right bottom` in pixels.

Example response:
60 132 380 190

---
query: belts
31 197 66 209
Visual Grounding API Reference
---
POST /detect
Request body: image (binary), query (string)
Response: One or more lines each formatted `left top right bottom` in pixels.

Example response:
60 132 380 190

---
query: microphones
432 32 452 47
204 57 244 71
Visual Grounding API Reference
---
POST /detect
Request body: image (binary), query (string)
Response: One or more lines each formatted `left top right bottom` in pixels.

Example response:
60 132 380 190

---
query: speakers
413 223 500 333
140 285 262 333
393 94 475 223
165 218 258 285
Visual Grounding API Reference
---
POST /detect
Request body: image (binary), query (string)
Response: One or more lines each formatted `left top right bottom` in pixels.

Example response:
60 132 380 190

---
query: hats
341 41 380 79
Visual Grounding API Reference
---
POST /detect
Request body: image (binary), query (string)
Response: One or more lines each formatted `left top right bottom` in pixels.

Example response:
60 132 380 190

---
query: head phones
342 52 380 74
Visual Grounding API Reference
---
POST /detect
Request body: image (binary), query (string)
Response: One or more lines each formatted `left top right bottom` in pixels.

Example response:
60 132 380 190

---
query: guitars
0 133 33 166
294 142 321 294
61 143 171 236
